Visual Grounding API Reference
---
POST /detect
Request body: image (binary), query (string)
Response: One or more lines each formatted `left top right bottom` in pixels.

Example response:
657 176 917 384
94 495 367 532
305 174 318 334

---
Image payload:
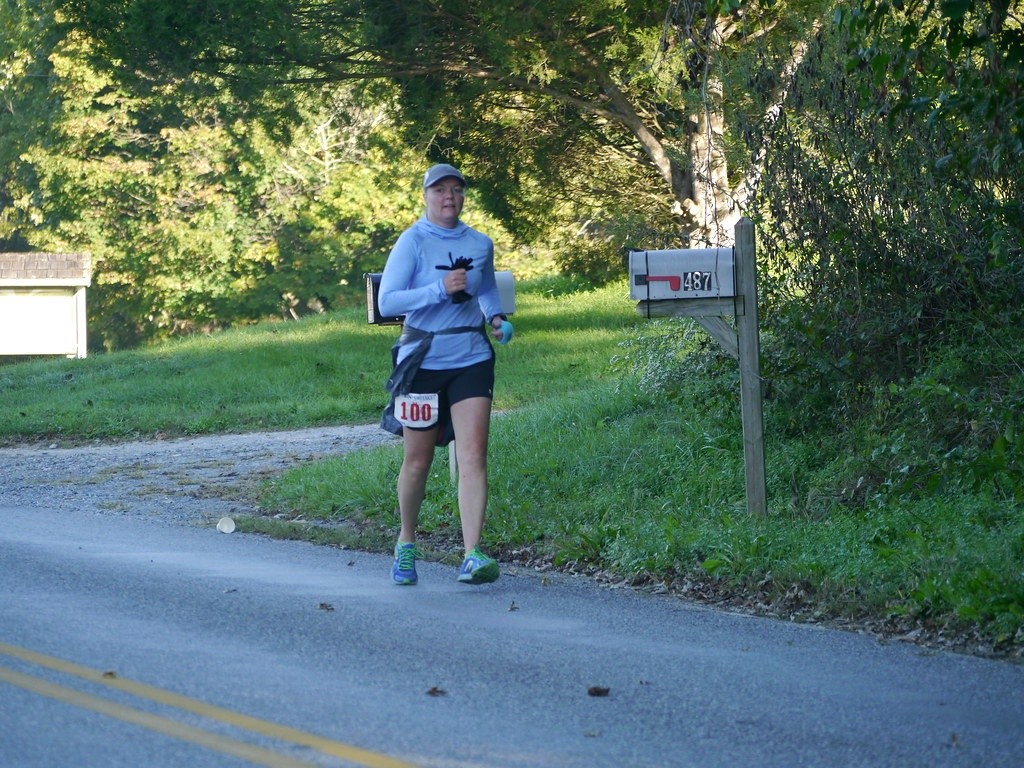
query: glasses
426 182 466 196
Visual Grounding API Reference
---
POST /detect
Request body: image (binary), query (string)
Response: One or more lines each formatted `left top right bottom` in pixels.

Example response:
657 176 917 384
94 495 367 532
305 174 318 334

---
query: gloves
435 252 474 304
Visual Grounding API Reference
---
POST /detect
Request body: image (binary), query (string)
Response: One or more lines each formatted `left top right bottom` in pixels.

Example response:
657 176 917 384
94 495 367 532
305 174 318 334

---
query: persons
378 164 513 586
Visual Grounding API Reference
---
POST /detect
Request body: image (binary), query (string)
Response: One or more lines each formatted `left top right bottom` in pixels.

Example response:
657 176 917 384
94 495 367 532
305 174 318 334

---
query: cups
216 517 235 533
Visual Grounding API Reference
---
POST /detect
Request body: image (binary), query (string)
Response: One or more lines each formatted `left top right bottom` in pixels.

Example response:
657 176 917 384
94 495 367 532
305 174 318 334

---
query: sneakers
458 544 500 585
390 538 417 585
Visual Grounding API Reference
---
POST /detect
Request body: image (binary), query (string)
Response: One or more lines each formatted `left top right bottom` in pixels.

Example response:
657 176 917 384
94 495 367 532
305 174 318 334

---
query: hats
423 163 466 189
